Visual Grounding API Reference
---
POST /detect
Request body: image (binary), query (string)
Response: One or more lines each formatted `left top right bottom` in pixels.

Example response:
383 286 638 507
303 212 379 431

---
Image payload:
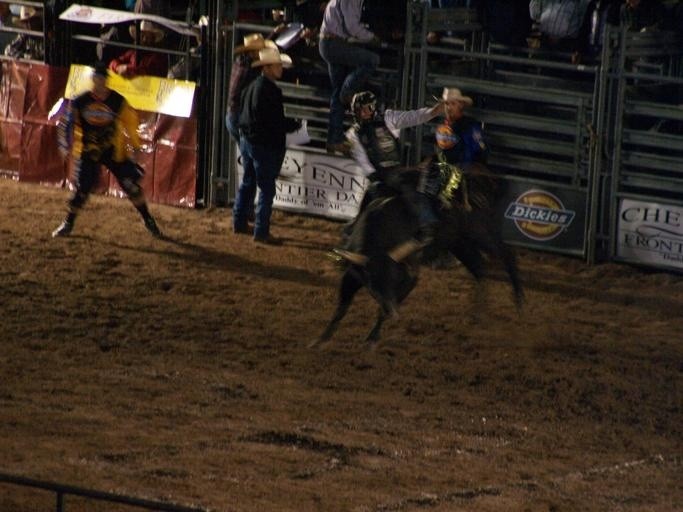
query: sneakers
326 142 349 153
235 206 283 246
52 221 72 238
144 218 160 237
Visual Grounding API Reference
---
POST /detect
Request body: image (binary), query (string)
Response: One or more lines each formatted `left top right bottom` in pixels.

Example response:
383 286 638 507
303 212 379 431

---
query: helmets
352 91 377 112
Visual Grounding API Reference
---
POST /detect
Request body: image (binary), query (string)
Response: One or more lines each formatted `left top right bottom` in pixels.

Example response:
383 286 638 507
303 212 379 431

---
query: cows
305 183 527 355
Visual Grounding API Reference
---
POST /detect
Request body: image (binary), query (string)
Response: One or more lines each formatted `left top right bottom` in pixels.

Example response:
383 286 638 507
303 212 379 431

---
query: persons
322 90 448 268
2 0 215 87
432 86 488 178
49 64 163 238
230 48 292 247
261 2 682 163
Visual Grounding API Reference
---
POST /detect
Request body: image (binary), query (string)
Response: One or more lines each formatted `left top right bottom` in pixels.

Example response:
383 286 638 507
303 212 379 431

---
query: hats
93 60 107 76
12 6 42 26
234 33 293 68
129 20 164 43
431 87 473 106
